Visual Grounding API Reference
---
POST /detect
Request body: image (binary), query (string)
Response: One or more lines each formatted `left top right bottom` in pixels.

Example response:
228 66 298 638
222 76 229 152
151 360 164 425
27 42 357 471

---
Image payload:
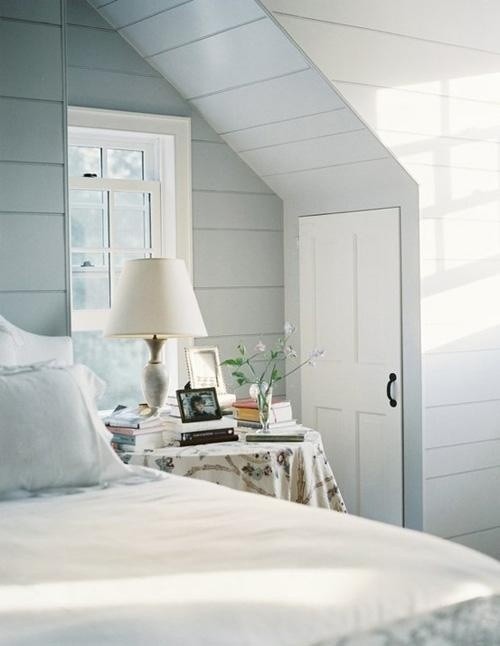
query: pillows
0 359 168 504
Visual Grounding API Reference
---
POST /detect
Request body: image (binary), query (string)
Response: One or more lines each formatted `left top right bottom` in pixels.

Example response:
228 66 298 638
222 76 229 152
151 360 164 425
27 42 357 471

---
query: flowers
217 317 327 419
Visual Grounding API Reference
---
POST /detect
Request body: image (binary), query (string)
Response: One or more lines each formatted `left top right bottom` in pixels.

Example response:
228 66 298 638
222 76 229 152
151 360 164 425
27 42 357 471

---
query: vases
252 389 276 435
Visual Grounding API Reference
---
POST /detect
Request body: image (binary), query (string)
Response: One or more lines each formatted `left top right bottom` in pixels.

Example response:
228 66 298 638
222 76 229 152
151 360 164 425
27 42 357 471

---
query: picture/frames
174 385 224 424
182 341 226 399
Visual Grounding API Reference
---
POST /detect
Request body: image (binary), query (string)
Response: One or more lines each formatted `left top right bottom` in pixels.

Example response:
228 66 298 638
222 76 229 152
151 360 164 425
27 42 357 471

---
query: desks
109 425 351 517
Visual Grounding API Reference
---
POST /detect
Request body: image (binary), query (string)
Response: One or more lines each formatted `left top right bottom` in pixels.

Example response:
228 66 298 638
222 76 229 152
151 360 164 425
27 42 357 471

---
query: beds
0 315 499 646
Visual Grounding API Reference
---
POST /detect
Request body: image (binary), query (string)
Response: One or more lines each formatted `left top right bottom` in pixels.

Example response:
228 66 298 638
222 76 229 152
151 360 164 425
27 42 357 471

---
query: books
103 400 305 453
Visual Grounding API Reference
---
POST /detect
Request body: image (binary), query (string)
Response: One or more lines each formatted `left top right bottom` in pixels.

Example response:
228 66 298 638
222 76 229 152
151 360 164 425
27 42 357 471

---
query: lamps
102 255 213 422
